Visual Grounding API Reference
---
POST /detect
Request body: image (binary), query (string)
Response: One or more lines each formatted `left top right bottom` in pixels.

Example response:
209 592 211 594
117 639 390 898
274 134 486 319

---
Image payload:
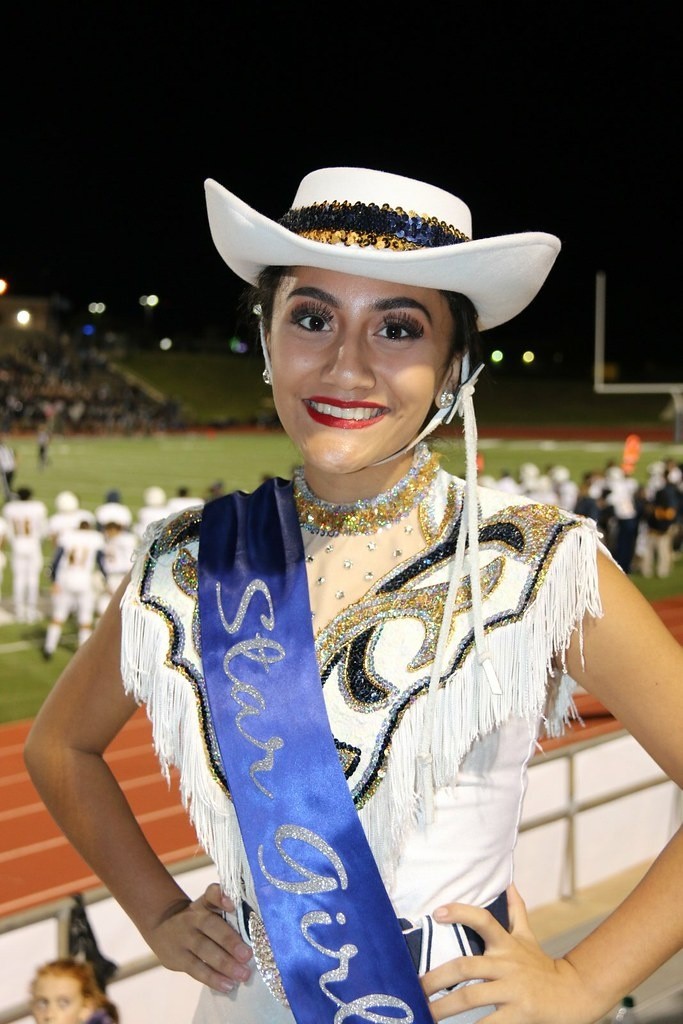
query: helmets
518 462 682 508
54 485 166 525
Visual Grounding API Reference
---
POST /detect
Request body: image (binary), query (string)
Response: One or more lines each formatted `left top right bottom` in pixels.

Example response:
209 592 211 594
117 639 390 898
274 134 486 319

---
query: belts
241 889 509 982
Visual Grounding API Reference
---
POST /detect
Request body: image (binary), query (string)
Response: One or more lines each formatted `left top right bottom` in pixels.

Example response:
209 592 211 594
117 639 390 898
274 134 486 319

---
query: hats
204 166 562 332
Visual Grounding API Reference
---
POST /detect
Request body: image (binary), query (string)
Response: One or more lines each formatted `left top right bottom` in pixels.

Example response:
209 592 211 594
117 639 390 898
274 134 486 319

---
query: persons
30 959 119 1024
0 346 683 662
23 167 683 1024
